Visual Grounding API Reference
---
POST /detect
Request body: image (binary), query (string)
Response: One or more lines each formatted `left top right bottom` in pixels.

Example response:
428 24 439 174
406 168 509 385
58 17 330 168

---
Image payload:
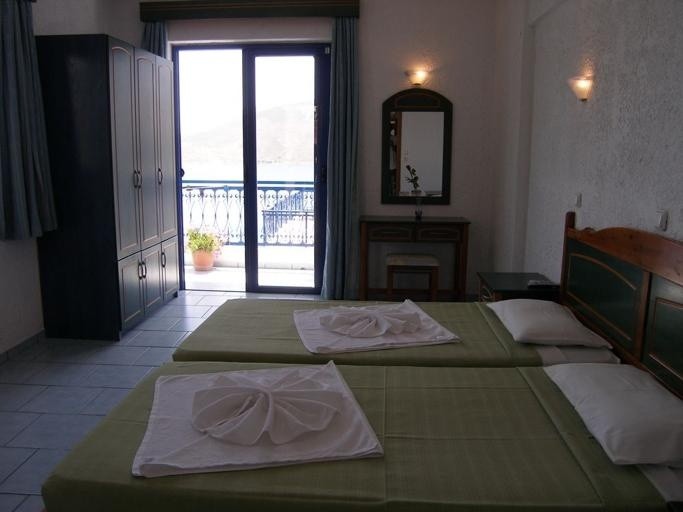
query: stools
386 253 441 300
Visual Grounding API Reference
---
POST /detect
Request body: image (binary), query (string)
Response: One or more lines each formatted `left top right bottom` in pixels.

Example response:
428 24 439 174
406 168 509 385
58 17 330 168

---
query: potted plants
187 229 223 271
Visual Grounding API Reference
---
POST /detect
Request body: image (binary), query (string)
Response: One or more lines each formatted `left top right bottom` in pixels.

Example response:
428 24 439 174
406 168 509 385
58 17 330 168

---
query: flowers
406 164 420 189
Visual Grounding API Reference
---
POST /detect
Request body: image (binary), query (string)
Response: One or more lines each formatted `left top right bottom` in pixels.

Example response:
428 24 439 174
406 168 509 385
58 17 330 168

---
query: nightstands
477 272 558 302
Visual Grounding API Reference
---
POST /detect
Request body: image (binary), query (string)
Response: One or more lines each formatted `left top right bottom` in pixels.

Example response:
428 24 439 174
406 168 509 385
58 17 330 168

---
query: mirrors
381 89 452 206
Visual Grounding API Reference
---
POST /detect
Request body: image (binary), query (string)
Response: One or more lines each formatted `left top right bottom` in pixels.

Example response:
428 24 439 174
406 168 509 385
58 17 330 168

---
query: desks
357 216 471 300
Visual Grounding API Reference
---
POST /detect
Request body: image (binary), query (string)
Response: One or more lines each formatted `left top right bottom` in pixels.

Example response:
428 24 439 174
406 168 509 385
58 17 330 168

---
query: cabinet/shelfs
29 35 182 340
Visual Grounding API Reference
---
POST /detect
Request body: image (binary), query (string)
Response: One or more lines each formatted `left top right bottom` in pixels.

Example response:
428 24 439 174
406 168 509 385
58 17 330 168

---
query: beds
43 235 683 511
173 211 652 368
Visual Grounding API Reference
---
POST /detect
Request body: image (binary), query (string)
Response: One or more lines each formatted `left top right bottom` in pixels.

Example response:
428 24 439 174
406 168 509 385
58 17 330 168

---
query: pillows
543 363 683 466
486 299 613 348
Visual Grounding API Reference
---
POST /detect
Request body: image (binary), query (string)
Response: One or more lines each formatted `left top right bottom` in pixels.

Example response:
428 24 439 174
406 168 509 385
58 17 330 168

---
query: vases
410 186 421 196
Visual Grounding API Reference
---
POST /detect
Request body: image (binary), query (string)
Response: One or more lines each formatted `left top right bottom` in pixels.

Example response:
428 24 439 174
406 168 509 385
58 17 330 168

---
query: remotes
526 279 560 288
